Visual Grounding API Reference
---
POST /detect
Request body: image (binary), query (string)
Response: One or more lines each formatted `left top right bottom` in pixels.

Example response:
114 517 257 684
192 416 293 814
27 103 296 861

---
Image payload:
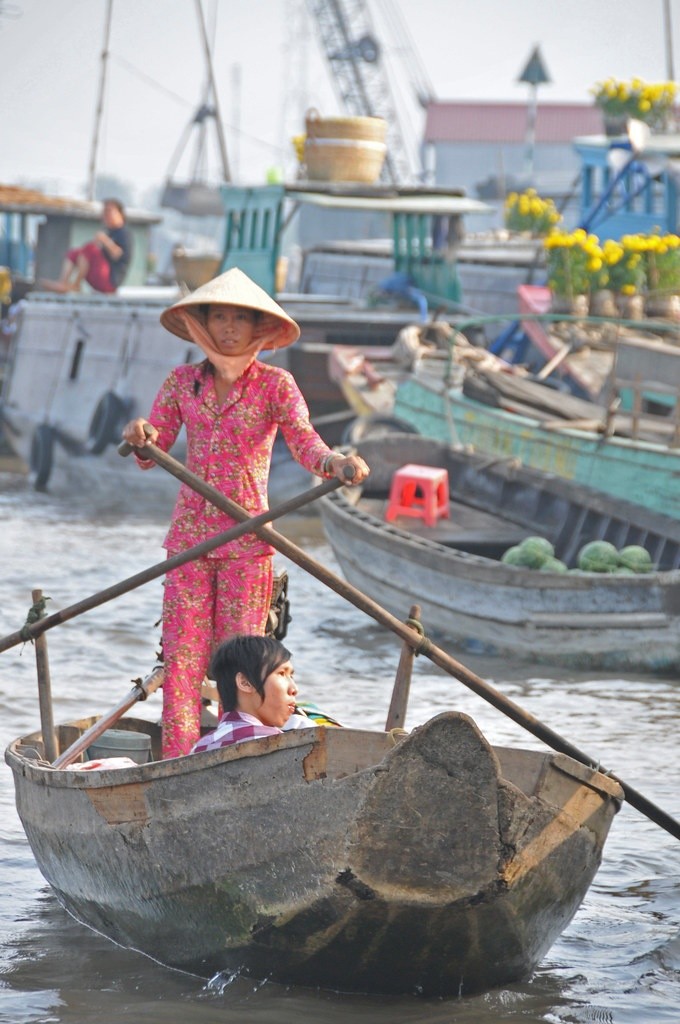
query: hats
158 266 301 352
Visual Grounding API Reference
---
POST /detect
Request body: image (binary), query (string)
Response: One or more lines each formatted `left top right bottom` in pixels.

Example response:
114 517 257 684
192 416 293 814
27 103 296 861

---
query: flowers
596 80 676 122
503 188 680 294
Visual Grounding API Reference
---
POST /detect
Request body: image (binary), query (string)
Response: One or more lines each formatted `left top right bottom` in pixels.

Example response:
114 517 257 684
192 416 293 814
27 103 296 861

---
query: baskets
172 248 219 290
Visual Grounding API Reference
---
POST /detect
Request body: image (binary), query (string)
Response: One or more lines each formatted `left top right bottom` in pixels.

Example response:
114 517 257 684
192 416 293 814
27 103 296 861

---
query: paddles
116 421 680 842
0 465 356 656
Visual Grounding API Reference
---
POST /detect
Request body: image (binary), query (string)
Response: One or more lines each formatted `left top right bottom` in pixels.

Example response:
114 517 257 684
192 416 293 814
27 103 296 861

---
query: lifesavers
87 391 122 454
30 426 54 488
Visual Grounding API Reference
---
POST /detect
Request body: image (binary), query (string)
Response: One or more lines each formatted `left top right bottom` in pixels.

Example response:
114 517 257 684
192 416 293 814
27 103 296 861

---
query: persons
190 636 297 753
122 268 371 760
40 201 132 293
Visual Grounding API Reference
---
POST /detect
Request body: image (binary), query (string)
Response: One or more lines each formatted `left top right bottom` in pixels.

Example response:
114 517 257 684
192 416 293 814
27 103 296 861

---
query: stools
385 464 449 528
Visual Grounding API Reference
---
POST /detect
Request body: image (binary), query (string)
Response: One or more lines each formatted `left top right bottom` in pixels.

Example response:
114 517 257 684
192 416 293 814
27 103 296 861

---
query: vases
508 230 533 239
605 121 627 136
535 230 546 240
552 289 680 322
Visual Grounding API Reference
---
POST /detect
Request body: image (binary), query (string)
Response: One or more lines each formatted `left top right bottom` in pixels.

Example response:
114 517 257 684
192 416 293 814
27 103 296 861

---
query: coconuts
502 537 653 575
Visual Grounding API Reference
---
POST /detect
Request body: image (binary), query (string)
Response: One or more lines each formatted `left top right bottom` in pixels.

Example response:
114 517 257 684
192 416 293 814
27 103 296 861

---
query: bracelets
326 454 334 475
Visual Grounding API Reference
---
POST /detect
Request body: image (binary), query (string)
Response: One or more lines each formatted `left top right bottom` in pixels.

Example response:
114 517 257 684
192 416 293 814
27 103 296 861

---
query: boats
0 0 680 526
310 430 680 684
4 706 629 1007
